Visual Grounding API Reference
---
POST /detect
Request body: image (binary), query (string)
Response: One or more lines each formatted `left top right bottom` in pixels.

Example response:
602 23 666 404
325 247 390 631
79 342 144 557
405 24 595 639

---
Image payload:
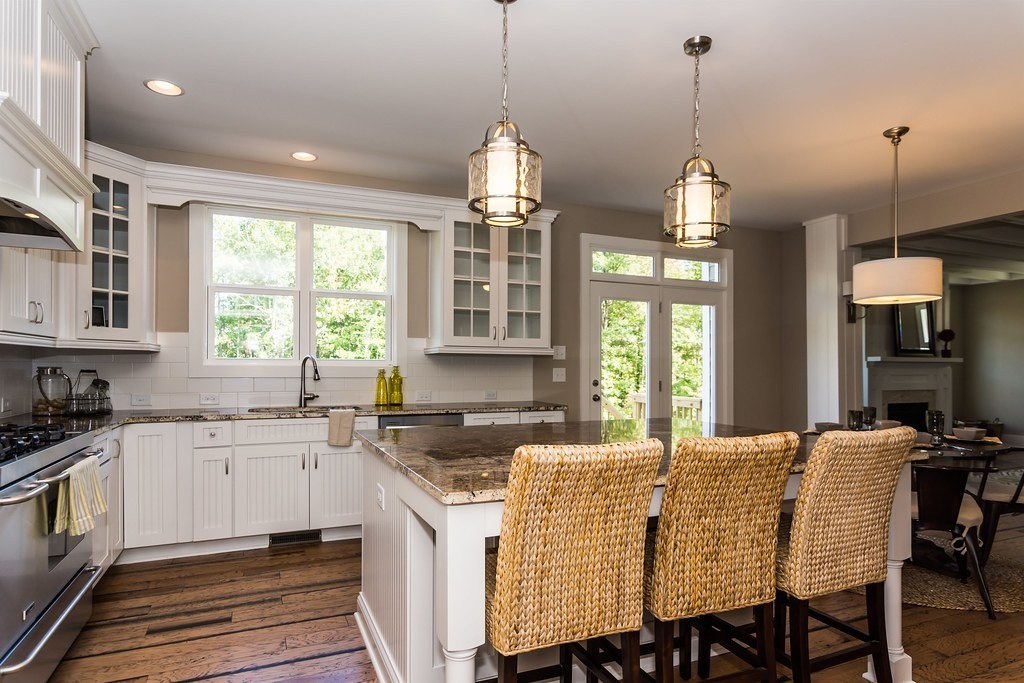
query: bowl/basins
875 420 902 430
915 431 933 443
815 422 843 431
952 427 987 440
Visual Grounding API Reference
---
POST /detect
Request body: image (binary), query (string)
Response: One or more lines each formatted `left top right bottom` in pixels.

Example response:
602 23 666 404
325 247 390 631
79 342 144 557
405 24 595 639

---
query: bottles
374 368 388 406
32 367 72 415
82 379 110 411
388 365 403 405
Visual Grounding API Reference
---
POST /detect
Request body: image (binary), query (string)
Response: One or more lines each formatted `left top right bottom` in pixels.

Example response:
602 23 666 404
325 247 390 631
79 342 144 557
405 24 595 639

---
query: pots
954 417 1004 442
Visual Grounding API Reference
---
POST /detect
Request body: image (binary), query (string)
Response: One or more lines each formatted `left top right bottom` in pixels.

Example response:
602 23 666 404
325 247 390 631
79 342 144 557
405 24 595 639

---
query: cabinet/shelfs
0 0 160 352
423 208 554 356
90 414 380 589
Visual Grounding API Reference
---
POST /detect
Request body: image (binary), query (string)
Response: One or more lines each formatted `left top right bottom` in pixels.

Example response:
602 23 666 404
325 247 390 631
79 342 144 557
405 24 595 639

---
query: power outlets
415 390 430 401
199 393 219 405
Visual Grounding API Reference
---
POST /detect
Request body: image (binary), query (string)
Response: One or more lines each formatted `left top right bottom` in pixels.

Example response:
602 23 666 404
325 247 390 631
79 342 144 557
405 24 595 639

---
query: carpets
844 535 1024 613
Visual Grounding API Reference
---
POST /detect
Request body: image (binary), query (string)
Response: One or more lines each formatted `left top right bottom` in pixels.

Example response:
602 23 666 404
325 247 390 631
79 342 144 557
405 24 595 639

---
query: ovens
0 446 104 683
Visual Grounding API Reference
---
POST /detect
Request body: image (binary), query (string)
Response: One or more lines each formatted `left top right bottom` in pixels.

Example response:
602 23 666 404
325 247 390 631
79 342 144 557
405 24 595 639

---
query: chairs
773 425 917 683
965 446 1024 574
911 464 997 621
481 437 665 683
639 431 800 683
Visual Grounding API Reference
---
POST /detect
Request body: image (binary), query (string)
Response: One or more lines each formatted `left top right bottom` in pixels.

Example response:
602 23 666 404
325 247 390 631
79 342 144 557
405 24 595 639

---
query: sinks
248 405 362 413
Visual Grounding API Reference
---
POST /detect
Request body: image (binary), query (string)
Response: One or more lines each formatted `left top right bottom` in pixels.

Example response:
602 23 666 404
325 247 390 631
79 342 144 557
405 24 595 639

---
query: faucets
300 356 321 407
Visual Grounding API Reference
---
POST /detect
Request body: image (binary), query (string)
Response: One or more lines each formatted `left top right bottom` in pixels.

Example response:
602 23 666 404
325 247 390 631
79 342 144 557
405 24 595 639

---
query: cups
862 407 876 431
847 410 862 431
925 410 944 436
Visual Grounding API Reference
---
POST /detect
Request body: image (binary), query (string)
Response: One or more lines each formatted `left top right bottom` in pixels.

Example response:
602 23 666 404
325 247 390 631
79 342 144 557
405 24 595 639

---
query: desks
907 432 1010 583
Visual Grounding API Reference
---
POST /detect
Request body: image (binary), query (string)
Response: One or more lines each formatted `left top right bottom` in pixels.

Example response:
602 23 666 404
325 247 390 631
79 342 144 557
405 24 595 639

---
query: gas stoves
0 422 94 487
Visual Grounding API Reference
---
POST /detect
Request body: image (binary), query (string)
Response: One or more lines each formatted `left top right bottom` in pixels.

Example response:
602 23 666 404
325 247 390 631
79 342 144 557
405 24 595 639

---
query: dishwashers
379 413 464 430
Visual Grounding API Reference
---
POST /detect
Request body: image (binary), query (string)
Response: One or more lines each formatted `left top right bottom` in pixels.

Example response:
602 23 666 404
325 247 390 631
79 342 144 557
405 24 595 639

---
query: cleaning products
389 365 404 404
376 368 388 404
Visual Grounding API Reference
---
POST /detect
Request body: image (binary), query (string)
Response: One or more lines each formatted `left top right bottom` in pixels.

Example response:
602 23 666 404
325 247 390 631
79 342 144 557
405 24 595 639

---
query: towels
328 409 355 447
54 456 110 536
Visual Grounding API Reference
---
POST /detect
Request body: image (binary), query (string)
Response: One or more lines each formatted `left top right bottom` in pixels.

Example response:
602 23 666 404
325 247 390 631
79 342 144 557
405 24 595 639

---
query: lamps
663 34 731 249
467 0 541 227
852 126 944 306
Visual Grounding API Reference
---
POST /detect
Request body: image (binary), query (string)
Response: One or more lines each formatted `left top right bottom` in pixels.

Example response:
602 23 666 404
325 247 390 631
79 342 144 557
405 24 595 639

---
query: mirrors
892 300 937 358
